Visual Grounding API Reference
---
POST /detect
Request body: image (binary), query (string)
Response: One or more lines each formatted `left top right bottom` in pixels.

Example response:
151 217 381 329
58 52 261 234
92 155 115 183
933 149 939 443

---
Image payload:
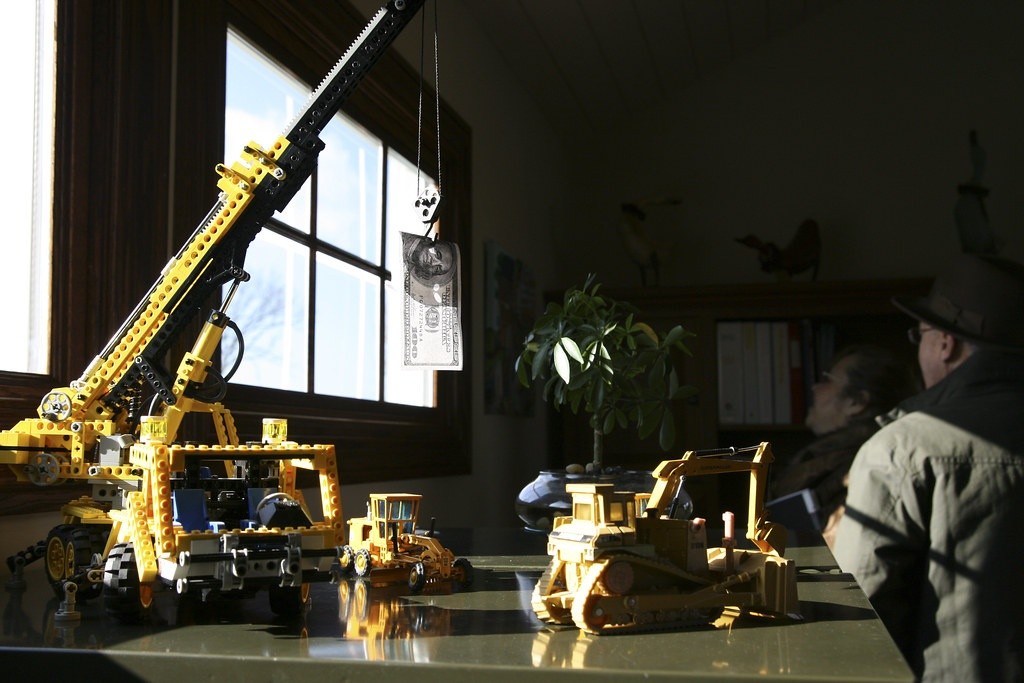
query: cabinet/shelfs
562 275 938 472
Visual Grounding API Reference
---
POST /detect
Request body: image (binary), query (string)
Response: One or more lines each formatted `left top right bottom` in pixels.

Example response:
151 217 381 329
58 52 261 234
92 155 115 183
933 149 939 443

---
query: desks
1 553 919 683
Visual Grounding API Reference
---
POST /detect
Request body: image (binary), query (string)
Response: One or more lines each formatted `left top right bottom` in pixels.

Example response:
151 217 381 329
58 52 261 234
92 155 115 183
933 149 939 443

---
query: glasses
908 327 935 343
819 370 847 386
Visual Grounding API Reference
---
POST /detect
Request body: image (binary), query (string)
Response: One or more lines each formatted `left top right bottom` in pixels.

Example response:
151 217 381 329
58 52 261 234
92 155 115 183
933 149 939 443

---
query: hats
890 254 1024 354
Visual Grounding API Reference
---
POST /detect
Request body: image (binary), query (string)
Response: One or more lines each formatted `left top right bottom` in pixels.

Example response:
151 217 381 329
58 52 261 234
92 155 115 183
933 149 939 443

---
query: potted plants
515 273 696 534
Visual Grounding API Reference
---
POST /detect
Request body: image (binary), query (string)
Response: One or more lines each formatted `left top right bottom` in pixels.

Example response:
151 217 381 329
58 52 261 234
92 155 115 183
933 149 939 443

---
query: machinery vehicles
337 578 451 662
337 492 473 591
0 0 447 623
530 625 791 675
531 441 804 636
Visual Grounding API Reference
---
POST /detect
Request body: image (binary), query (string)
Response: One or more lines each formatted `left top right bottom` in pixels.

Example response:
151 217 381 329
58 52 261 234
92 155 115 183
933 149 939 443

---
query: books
762 488 826 547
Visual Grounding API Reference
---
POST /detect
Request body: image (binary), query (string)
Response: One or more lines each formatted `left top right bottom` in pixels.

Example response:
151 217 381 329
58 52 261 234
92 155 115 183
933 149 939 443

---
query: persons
764 345 922 529
822 258 1024 683
